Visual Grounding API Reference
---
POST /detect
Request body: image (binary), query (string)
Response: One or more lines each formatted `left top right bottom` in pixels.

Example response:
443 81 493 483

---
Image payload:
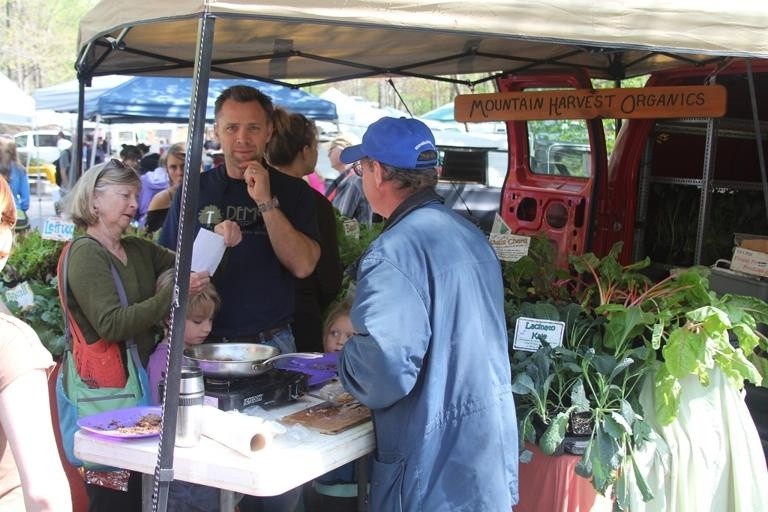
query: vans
12 130 72 163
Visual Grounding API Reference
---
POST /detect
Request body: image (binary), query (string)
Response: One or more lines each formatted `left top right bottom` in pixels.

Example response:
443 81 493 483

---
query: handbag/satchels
56 234 151 471
57 241 127 387
14 209 31 231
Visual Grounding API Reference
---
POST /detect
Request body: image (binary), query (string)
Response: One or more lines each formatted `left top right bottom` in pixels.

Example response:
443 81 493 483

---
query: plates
77 406 161 440
199 404 275 461
275 352 341 387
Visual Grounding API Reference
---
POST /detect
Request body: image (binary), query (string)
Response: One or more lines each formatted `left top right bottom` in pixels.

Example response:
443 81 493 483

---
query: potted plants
517 375 633 454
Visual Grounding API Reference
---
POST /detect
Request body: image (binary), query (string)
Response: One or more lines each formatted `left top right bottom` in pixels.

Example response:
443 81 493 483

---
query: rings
253 169 256 173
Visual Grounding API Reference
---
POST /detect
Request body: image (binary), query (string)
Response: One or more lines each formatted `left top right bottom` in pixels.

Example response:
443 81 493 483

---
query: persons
147 267 220 405
0 172 74 512
265 107 339 353
323 138 372 230
56 158 243 512
311 300 374 500
138 151 171 230
0 136 30 237
338 116 520 512
120 144 141 175
158 86 323 356
54 144 73 218
136 143 150 159
147 141 187 234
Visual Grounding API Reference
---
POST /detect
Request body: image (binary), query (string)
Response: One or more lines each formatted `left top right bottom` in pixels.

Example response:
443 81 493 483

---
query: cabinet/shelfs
515 373 749 509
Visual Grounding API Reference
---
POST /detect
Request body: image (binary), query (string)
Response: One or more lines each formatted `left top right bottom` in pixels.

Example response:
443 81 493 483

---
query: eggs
250 434 266 452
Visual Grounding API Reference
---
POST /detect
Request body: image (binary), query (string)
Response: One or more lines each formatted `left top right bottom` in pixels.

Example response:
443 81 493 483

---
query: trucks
493 59 768 294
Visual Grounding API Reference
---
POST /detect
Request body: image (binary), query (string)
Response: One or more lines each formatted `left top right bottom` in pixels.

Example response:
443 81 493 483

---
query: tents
72 0 768 511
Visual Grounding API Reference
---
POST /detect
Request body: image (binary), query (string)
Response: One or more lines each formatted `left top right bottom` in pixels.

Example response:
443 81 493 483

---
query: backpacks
52 149 72 186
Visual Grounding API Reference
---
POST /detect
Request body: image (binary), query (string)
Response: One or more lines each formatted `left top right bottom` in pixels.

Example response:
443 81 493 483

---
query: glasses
97 159 128 180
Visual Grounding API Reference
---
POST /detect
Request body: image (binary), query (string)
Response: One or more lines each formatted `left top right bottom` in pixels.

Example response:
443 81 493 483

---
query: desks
74 359 375 512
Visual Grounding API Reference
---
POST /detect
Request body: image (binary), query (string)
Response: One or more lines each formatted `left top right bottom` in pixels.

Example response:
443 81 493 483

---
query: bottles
170 366 204 447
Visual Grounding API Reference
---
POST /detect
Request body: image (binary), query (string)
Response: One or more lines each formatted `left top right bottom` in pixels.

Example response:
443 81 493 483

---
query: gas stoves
204 368 309 412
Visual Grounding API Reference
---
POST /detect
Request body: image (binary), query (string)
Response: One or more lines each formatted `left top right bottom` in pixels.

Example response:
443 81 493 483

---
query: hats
329 136 352 149
339 116 440 170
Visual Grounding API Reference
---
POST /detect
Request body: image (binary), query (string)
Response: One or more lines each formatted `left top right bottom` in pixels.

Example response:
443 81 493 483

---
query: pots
184 343 326 376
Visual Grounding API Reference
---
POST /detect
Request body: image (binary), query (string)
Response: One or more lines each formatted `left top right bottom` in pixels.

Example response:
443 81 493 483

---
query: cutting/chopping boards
282 398 373 436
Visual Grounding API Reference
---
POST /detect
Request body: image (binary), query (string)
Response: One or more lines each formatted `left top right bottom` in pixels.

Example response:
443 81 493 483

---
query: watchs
257 195 280 213
